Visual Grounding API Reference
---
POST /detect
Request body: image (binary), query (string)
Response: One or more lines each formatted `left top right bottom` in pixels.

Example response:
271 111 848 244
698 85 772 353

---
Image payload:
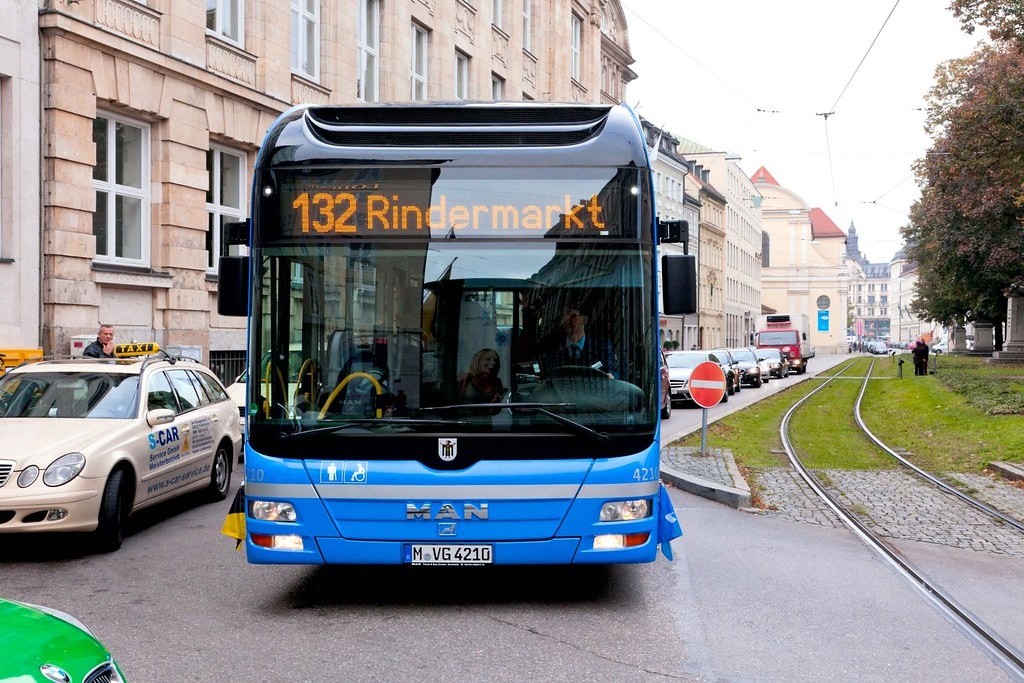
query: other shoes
923 373 927 375
919 374 924 376
915 374 918 376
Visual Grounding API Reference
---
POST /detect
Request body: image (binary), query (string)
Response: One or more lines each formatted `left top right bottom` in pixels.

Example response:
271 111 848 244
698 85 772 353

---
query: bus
239 100 665 572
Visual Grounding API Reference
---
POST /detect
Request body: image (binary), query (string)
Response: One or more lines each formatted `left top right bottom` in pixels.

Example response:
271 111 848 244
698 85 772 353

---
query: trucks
753 313 816 374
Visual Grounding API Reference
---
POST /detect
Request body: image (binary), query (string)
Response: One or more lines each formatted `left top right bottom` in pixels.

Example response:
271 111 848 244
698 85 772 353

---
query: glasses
569 312 583 320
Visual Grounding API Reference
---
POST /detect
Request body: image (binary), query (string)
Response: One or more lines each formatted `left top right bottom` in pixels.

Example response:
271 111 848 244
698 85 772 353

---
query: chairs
331 350 388 420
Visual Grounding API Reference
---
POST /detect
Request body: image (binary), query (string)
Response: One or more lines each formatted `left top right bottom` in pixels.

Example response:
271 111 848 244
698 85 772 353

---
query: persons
853 341 857 353
919 338 929 375
537 300 619 382
911 341 927 376
858 341 862 353
83 324 116 359
457 348 509 415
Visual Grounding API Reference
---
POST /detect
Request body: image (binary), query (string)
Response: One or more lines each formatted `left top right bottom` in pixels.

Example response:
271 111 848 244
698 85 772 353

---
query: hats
916 341 922 345
568 291 593 315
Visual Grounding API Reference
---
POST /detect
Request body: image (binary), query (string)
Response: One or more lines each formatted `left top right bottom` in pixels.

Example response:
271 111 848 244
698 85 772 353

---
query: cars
754 355 770 381
847 334 978 355
705 349 740 396
657 346 671 417
0 595 132 683
723 347 765 388
662 352 729 404
214 342 314 426
753 349 789 379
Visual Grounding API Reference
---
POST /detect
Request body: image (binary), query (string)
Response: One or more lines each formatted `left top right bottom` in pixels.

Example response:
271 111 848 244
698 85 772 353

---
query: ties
570 344 580 366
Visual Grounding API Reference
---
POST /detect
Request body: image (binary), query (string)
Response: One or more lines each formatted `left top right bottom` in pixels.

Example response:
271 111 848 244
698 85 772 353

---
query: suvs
0 359 238 551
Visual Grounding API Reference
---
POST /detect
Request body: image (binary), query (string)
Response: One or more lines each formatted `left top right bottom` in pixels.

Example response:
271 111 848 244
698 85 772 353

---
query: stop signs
688 361 726 410
